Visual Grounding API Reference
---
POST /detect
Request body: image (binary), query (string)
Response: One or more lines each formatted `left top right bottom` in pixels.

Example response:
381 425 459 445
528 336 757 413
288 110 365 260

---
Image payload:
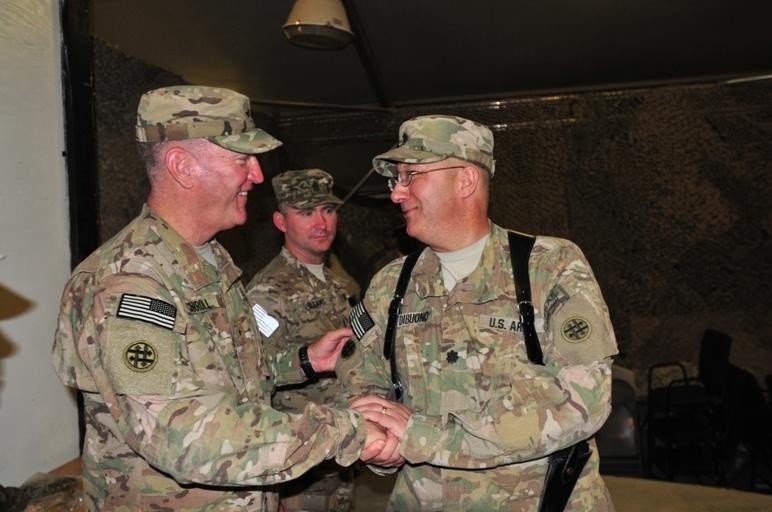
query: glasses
385 163 463 192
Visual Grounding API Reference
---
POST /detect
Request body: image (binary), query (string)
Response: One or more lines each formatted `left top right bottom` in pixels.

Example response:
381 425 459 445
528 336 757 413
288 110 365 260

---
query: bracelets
299 342 318 385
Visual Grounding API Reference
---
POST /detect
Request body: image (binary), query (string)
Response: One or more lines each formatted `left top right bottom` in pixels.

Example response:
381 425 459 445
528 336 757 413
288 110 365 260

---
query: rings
382 405 388 414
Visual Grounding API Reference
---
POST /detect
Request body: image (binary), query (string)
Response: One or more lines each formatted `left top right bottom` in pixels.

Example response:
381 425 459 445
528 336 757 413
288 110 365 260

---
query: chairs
643 323 735 486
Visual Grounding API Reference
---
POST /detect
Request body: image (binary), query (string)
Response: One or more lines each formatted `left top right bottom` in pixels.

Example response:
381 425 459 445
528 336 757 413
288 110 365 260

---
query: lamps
275 1 359 54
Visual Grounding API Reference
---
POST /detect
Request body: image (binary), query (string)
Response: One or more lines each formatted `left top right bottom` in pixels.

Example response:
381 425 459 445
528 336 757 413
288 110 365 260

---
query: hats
271 169 346 209
371 112 498 177
135 84 284 155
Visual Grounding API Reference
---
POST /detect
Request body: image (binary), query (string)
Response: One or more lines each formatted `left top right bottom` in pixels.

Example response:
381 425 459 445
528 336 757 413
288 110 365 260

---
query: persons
331 112 624 512
242 165 369 512
50 86 392 511
